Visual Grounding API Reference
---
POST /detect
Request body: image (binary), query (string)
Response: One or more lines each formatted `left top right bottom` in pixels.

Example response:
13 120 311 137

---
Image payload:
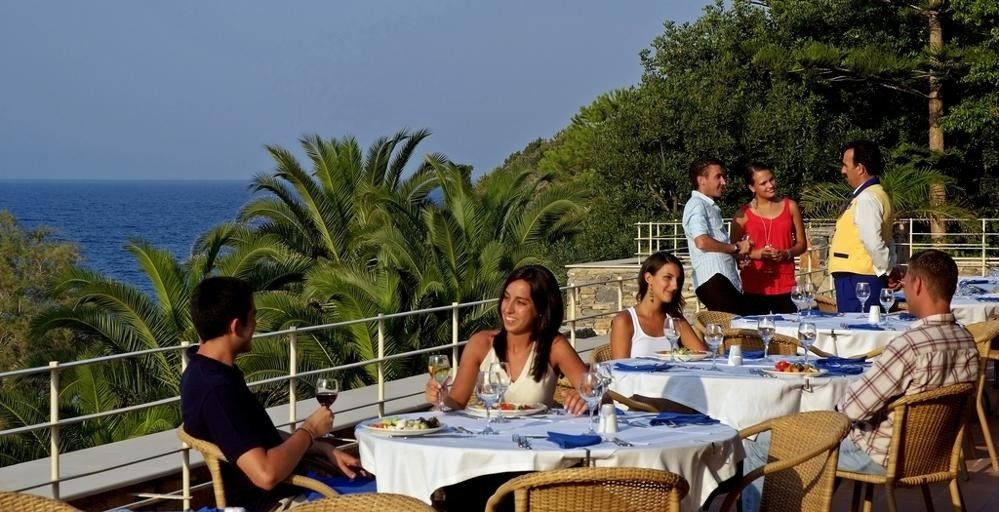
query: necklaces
752 199 774 248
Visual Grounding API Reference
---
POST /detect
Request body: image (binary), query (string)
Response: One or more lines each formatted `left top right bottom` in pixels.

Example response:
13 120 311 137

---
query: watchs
735 243 740 254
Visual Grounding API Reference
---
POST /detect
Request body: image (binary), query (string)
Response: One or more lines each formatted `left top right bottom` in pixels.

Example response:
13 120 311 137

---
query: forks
512 432 532 456
748 366 776 382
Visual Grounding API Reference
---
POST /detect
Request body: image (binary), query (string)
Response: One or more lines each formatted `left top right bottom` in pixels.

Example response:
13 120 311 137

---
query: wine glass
428 356 453 416
855 282 870 318
478 362 514 435
577 358 617 437
878 287 895 322
757 317 777 362
662 317 686 364
795 323 817 368
741 233 754 266
704 322 723 373
762 244 776 276
790 282 817 320
311 378 339 438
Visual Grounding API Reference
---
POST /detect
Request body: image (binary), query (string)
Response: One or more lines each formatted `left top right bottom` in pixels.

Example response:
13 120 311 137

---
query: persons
424 262 610 512
730 162 808 315
827 248 981 496
608 250 713 360
681 156 756 316
176 277 378 512
826 138 903 313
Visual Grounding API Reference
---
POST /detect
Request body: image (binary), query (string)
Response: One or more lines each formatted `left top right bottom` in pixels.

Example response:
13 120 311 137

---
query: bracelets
786 249 792 260
295 428 314 446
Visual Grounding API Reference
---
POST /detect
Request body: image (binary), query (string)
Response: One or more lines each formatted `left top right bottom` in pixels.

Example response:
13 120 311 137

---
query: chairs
835 382 974 510
697 411 852 508
465 371 579 410
587 278 999 440
1 490 77 509
288 493 431 509
487 465 690 509
177 421 362 510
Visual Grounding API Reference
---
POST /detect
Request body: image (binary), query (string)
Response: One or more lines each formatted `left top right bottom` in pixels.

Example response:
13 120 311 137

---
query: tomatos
776 359 788 370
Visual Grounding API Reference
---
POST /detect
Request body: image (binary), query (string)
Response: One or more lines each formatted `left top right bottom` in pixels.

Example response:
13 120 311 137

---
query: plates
358 415 447 436
656 349 714 361
762 364 828 380
468 398 550 419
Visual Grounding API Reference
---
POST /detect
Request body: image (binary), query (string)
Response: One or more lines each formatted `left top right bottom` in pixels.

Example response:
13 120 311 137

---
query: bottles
728 344 743 368
868 306 882 324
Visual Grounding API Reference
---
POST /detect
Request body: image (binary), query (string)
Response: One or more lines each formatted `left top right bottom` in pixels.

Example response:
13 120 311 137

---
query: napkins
545 430 601 450
